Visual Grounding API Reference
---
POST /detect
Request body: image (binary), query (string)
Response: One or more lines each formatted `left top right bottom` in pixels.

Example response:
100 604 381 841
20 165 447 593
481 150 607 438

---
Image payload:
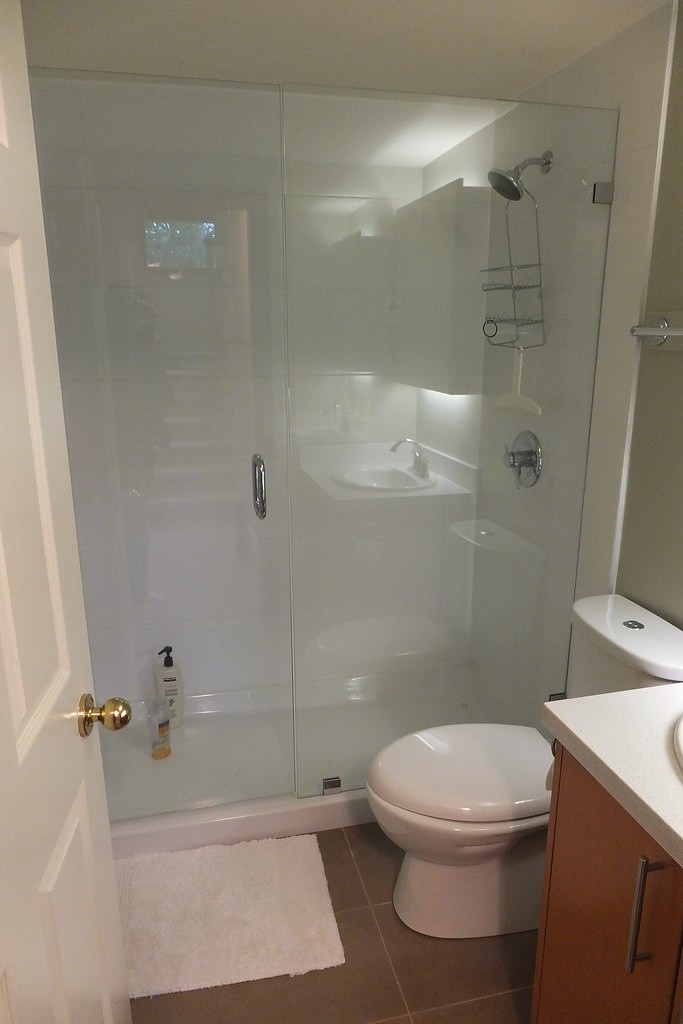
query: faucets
391 438 432 478
323 403 353 434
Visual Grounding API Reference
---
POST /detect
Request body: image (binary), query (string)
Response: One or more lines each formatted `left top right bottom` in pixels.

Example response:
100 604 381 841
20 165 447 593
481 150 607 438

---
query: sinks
296 424 361 442
330 464 440 491
674 711 683 769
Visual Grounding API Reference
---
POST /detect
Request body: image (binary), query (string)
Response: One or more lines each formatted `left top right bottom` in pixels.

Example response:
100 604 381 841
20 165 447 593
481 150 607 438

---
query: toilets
358 517 537 722
368 593 683 936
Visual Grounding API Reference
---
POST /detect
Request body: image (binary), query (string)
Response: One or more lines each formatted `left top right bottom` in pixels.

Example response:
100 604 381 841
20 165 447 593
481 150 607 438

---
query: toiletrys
153 645 185 729
151 698 172 760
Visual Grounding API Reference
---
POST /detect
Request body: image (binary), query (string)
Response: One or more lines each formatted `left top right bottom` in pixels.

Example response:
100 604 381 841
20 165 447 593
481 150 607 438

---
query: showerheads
488 167 524 200
